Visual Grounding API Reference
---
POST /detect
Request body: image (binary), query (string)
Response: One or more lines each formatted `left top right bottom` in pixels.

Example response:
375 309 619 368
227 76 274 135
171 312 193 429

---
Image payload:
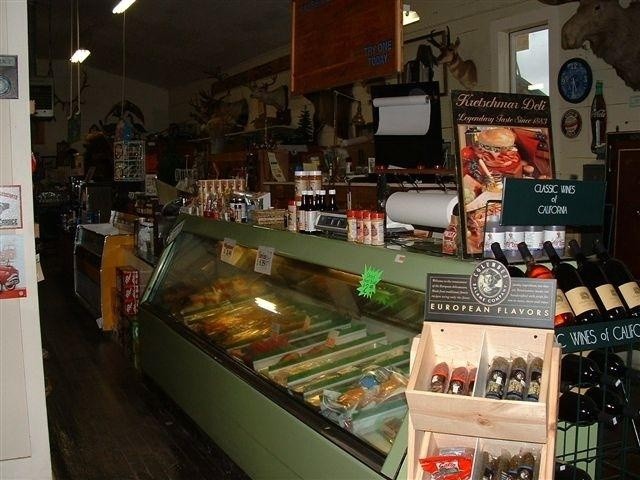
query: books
112 263 141 372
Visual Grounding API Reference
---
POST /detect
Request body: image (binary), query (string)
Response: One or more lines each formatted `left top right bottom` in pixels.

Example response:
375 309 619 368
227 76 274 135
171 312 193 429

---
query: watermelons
313 212 414 237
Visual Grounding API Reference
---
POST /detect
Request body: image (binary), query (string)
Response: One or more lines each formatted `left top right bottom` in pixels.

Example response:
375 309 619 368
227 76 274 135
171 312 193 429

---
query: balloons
556 55 595 105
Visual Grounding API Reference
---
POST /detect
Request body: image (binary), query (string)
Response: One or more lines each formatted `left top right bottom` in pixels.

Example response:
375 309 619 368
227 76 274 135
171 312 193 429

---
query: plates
480 193 640 480
182 149 289 207
140 212 604 479
404 315 563 480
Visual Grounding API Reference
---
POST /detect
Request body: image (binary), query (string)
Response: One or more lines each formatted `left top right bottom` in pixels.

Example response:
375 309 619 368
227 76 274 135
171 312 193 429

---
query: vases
199 112 240 136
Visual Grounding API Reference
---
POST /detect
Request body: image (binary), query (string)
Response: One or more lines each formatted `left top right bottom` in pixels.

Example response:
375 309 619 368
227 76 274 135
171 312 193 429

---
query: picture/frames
71 220 134 333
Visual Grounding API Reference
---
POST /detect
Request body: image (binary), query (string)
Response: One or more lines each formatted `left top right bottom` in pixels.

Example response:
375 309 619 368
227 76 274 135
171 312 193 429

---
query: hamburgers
475 127 517 168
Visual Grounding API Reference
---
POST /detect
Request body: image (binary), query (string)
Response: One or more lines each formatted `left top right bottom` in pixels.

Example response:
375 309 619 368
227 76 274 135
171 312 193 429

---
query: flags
211 135 224 155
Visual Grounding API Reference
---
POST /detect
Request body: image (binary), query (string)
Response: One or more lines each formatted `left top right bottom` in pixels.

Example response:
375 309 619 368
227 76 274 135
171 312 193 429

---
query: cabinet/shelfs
554 459 592 480
489 238 638 326
300 189 337 236
590 80 608 152
559 351 640 429
484 212 566 259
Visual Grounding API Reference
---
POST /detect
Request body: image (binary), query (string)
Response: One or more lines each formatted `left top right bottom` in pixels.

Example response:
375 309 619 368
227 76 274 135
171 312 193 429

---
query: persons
479 269 501 296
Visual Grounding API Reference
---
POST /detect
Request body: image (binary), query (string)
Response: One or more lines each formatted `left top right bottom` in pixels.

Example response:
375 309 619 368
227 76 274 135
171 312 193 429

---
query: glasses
398 4 422 26
